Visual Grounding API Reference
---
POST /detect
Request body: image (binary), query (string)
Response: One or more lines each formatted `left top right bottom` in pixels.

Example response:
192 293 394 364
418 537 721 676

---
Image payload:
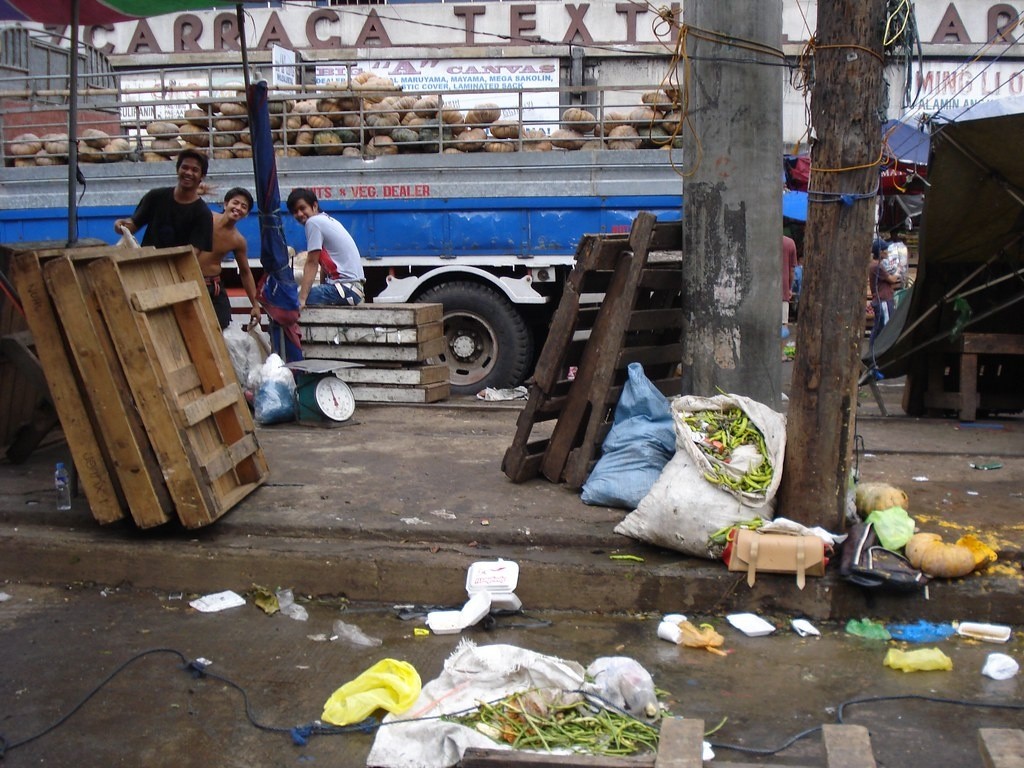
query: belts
204 276 221 297
334 282 364 299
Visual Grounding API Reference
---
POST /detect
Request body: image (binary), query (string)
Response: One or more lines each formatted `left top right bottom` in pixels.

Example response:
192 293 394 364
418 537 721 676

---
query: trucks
0 27 810 394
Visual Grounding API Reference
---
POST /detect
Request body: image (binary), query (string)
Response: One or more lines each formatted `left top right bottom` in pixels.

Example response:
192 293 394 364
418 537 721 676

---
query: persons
781 231 900 362
115 149 363 337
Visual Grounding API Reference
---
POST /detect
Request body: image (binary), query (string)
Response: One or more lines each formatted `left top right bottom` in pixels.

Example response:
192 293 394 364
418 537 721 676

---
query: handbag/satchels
724 523 835 591
839 522 933 601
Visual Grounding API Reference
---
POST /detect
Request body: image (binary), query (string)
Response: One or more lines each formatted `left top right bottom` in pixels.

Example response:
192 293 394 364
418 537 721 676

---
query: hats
872 239 893 252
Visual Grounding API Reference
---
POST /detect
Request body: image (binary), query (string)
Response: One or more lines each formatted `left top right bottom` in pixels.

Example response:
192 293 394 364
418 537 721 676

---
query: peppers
610 409 772 562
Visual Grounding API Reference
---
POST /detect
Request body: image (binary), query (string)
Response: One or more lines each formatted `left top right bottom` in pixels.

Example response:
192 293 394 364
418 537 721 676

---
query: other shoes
782 357 792 362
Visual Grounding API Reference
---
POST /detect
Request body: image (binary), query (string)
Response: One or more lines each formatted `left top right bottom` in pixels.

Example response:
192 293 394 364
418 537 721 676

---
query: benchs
929 332 1024 421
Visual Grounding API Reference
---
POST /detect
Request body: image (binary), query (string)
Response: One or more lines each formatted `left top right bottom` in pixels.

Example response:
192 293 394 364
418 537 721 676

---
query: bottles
55 463 71 510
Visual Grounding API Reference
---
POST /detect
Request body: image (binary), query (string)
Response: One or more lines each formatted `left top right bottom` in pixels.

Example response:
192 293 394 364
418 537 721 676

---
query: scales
277 358 365 430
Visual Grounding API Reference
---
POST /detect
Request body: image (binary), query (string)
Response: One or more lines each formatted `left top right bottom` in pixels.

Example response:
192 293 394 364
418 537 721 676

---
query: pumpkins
853 481 998 577
10 72 684 167
